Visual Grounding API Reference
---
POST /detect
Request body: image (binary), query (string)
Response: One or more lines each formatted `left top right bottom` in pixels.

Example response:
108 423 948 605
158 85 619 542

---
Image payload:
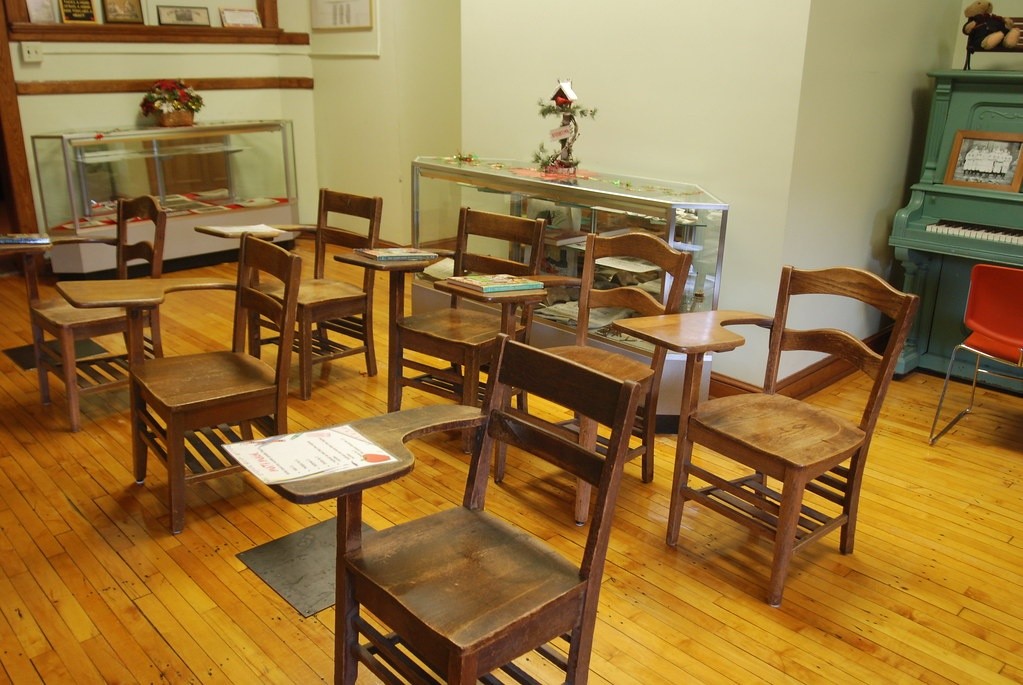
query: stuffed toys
962 0 1020 50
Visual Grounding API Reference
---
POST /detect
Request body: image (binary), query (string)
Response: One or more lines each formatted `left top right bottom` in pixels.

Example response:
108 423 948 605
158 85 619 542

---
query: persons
963 145 1013 180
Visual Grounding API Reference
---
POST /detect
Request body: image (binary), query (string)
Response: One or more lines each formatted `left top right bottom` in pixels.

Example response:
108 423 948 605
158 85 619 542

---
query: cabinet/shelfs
35 117 302 274
410 156 728 429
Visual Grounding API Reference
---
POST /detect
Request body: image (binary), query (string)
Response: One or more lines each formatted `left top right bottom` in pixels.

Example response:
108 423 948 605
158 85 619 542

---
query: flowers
141 79 205 117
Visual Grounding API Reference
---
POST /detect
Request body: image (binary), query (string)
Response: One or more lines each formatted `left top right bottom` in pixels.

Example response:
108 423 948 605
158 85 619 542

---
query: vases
160 106 195 126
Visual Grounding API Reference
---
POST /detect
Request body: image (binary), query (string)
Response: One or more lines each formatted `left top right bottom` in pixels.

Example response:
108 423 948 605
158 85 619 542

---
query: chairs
387 208 546 455
496 231 694 525
35 194 169 430
330 333 640 685
669 264 920 609
129 233 304 534
928 265 1023 446
242 186 383 399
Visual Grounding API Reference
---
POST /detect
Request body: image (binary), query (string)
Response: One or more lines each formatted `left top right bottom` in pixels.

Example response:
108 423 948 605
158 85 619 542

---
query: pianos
881 67 1023 394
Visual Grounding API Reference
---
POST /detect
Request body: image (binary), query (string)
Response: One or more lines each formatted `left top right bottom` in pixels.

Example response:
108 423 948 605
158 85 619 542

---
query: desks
433 271 583 347
56 277 239 368
0 232 69 307
611 311 776 419
192 222 286 282
221 400 483 553
333 248 443 326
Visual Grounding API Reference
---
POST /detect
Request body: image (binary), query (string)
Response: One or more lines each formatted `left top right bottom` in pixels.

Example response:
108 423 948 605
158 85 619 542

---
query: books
446 274 544 293
582 221 631 237
0 232 51 244
544 229 588 247
353 248 438 261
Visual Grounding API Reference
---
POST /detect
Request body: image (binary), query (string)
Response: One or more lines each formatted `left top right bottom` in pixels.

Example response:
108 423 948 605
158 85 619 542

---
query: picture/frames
26 0 56 23
156 5 210 25
307 1 373 34
58 0 97 24
102 0 144 24
218 6 262 27
